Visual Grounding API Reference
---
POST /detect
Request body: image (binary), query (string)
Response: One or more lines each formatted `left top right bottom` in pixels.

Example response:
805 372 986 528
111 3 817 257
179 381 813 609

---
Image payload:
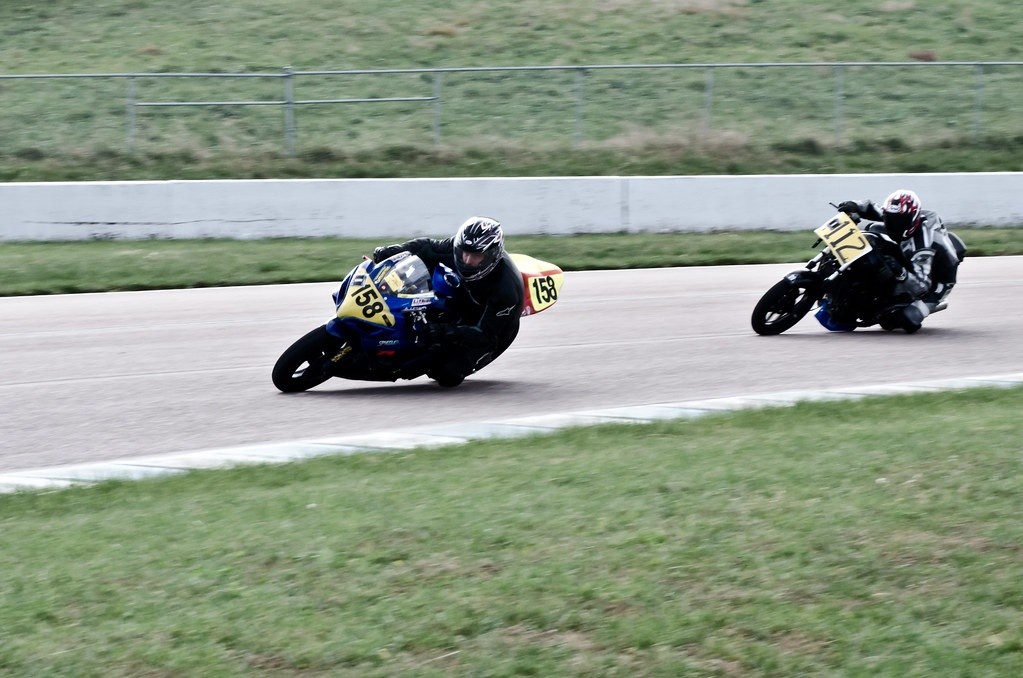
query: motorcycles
272 249 564 393
751 203 968 334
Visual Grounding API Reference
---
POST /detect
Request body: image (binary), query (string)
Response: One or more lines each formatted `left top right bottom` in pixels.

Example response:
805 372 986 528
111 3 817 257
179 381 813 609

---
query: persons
373 216 525 386
836 190 961 334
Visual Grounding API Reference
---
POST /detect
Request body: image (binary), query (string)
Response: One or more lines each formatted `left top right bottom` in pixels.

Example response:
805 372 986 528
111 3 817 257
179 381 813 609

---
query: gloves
838 201 859 214
883 254 903 278
373 247 392 264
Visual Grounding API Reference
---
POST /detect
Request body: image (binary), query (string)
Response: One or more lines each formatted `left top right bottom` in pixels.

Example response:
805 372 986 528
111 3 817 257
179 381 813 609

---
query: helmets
452 217 504 281
882 191 921 240
